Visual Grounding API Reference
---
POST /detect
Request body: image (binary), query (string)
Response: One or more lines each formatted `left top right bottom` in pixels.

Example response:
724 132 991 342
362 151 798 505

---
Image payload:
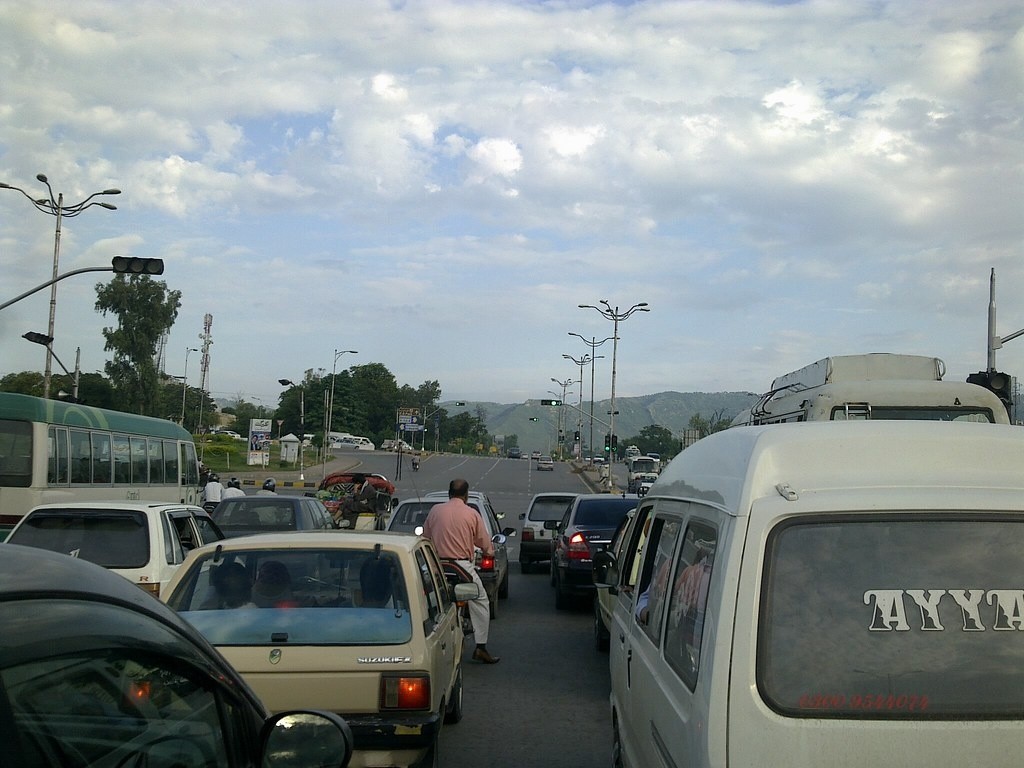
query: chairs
250 561 299 610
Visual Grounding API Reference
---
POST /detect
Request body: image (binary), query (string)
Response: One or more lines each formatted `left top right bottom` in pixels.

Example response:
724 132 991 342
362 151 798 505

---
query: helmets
209 473 220 483
263 478 276 492
228 478 240 489
414 452 418 456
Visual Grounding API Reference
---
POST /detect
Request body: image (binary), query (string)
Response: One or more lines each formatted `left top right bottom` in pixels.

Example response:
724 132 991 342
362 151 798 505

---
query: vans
590 351 1023 768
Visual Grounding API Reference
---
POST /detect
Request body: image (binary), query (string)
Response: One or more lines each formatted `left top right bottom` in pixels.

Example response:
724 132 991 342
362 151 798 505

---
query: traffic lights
605 435 617 453
541 400 562 406
111 255 164 276
575 431 580 440
529 418 539 422
976 370 1012 423
456 402 465 406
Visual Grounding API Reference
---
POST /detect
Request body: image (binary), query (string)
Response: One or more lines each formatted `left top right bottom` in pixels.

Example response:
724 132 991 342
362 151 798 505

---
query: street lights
278 378 305 483
180 348 198 428
547 290 652 470
0 173 122 401
329 349 359 436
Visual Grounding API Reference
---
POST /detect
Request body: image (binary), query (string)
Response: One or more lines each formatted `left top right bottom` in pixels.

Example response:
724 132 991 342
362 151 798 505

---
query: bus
628 453 660 494
625 448 639 465
0 392 209 542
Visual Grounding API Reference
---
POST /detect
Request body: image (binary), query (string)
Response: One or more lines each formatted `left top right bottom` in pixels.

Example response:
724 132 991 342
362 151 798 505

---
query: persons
330 473 378 526
250 478 282 525
251 435 262 451
203 475 225 508
419 479 501 663
650 543 673 639
636 583 651 623
672 546 716 666
391 440 394 452
413 454 419 468
359 557 395 609
222 479 247 516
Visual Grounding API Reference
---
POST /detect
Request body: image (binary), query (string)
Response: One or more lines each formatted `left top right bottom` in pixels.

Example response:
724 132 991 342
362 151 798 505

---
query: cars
218 430 412 454
517 489 654 652
0 472 518 768
634 474 658 493
508 447 555 472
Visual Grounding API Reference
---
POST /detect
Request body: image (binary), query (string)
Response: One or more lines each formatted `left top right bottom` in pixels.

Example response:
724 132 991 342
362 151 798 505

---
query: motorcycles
411 460 420 471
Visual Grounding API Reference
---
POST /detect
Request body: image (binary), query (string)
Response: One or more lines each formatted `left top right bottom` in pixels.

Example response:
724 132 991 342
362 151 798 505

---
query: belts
439 557 470 561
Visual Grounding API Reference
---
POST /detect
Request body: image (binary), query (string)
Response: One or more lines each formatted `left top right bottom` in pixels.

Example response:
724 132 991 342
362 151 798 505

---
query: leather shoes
472 648 500 664
